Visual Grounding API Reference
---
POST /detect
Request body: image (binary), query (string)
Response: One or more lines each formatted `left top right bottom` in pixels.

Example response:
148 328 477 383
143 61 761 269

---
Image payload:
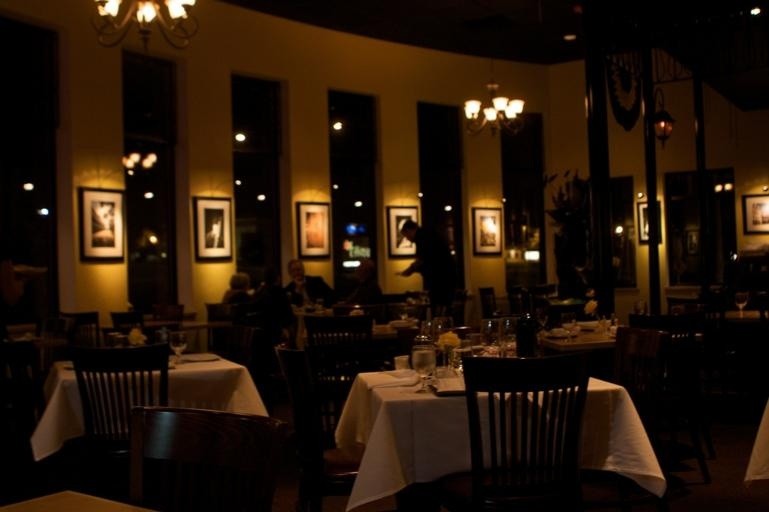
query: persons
223 257 381 378
393 219 458 328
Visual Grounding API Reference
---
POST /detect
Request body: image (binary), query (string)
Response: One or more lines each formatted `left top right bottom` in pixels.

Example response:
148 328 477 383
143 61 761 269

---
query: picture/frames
192 196 232 260
295 201 331 258
742 194 769 234
77 187 125 261
637 202 662 244
386 206 418 257
472 207 502 255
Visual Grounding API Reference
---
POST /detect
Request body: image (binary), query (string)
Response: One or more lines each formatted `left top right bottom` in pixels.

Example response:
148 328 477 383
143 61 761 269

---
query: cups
105 331 130 349
289 293 324 313
394 355 410 373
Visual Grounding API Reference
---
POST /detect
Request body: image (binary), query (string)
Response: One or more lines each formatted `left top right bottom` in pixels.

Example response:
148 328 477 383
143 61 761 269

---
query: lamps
83 0 203 49
646 87 673 148
461 56 529 137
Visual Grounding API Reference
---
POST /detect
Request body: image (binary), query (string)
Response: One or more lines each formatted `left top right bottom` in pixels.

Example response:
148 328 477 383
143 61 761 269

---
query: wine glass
734 292 748 316
412 317 512 392
420 290 428 304
170 331 188 367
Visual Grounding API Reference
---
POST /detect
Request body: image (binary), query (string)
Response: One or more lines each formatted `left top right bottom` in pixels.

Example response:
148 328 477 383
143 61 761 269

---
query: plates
389 318 418 328
182 353 219 361
546 328 580 338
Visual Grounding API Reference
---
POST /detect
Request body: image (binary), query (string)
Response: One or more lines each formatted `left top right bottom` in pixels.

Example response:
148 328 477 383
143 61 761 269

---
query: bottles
571 319 579 337
593 313 619 339
515 287 539 357
